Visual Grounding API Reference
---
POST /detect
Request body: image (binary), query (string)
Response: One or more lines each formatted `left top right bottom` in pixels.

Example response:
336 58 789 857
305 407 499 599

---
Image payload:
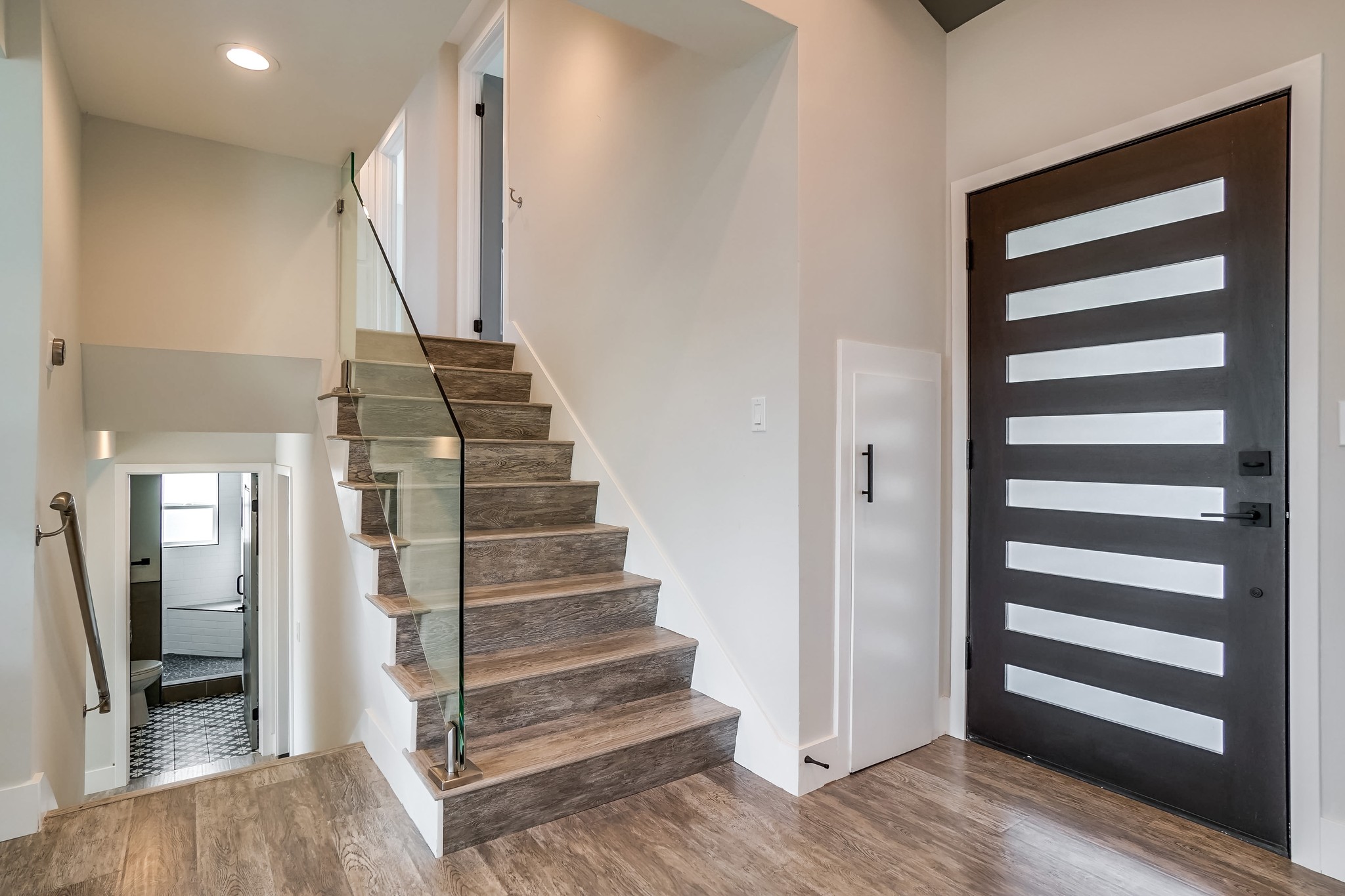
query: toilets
129 659 165 729
